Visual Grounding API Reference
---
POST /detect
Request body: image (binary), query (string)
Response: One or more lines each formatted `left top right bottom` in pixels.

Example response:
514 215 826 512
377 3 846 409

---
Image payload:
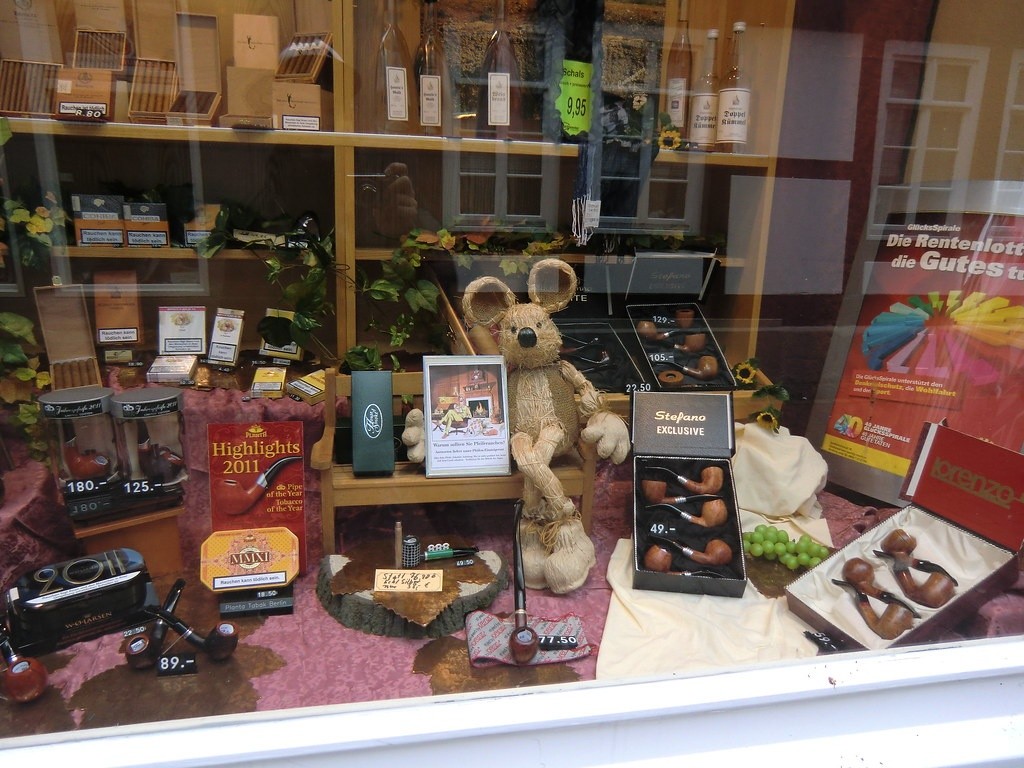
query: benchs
311 367 602 554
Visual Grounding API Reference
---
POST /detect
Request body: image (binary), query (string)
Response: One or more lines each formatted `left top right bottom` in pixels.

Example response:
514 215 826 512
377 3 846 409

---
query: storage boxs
787 504 1019 653
199 527 300 594
28 189 328 408
553 319 645 397
1 9 336 131
633 456 749 598
333 415 410 464
627 302 737 390
6 547 151 641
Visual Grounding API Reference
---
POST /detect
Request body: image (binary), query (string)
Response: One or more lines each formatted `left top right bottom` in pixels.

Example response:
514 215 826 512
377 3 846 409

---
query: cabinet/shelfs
1 0 796 423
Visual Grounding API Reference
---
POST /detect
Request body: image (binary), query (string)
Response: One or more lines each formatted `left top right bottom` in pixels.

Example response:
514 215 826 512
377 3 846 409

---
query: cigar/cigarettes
132 61 175 113
277 35 326 73
54 359 98 388
75 33 124 70
0 59 62 117
170 91 217 114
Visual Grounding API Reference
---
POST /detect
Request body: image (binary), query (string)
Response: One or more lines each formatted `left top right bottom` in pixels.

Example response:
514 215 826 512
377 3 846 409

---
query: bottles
688 29 720 152
414 0 454 137
718 22 753 152
375 0 417 134
476 0 526 140
665 18 691 138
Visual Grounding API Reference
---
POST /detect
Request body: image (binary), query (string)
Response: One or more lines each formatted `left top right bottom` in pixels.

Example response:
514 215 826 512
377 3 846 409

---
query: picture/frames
423 354 510 479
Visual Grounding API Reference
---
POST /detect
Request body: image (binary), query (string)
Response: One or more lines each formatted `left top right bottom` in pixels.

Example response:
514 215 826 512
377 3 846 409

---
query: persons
225 56 458 348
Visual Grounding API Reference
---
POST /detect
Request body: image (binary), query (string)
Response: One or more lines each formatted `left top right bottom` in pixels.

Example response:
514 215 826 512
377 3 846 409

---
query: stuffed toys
462 258 631 596
